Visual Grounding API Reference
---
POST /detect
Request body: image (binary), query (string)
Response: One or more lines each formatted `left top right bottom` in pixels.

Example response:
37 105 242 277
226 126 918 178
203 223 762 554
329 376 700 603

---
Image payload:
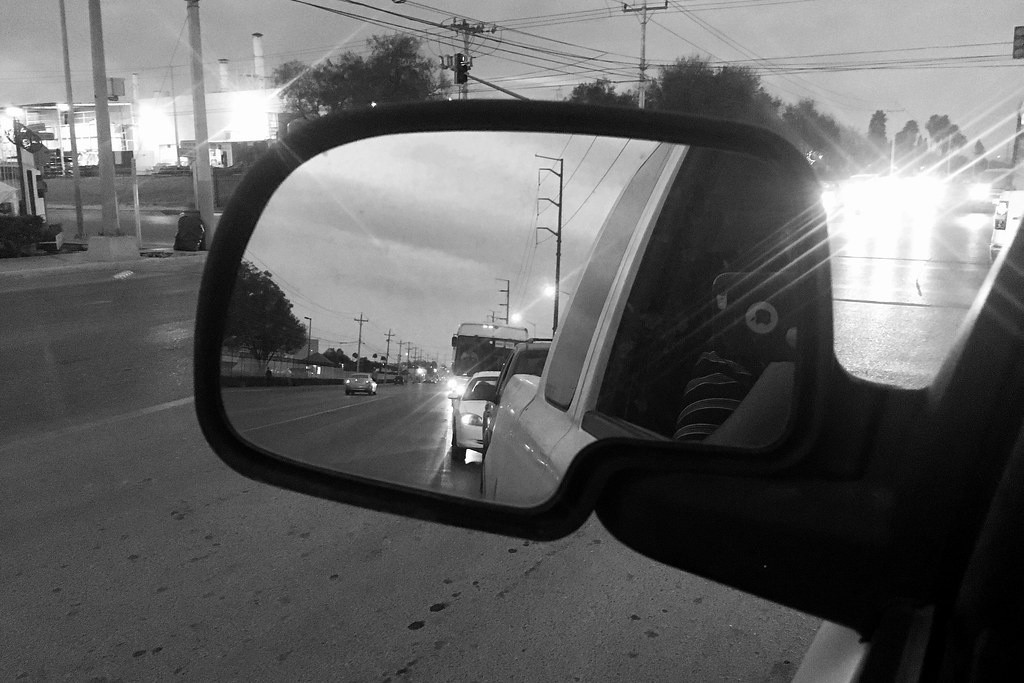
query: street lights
304 316 312 364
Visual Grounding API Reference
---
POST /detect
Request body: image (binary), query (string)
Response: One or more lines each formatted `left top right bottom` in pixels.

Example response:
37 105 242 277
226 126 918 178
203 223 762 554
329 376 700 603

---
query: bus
448 323 528 375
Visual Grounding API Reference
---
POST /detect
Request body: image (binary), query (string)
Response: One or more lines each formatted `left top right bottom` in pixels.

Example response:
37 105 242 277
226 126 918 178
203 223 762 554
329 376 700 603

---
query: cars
393 376 404 384
424 366 449 384
345 373 378 396
0 181 28 219
447 371 503 452
987 94 1024 259
818 157 1009 283
